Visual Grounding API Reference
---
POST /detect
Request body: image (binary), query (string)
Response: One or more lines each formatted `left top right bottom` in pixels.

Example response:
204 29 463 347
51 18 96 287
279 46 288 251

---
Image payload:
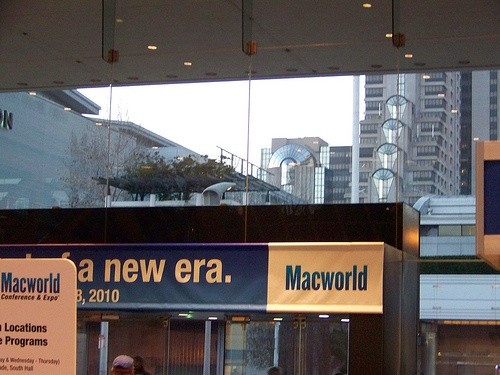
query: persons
109 355 136 375
330 348 349 375
268 367 282 375
133 356 150 375
